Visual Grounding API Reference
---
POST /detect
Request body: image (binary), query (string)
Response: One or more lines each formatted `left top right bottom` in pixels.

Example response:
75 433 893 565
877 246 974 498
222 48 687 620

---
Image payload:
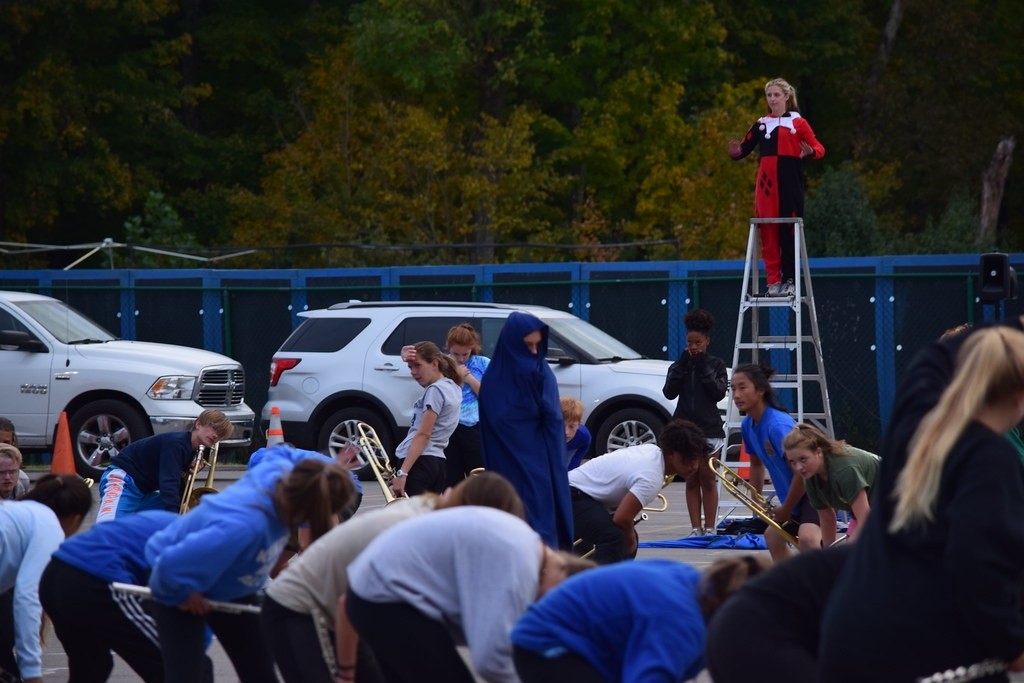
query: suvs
253 298 745 485
0 288 257 484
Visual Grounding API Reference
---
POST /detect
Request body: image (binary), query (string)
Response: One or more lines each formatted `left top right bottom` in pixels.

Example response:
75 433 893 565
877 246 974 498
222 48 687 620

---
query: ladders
714 217 846 531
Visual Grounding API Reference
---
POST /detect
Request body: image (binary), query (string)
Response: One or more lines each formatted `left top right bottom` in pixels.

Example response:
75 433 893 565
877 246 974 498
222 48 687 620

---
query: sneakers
767 282 781 296
779 284 794 296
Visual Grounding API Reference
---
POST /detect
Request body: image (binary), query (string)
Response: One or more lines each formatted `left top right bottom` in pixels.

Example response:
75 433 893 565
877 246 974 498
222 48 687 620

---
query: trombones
180 442 221 515
573 473 678 558
707 454 798 546
358 423 409 507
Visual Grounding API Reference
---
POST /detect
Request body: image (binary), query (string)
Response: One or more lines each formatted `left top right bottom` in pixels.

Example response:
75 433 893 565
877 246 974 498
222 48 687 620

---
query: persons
250 441 370 580
558 397 593 475
0 472 96 683
96 409 236 525
510 553 777 683
823 311 1024 683
568 419 709 568
393 341 463 499
444 324 493 489
38 510 215 683
704 541 856 683
663 309 729 541
144 442 362 683
346 506 598 683
783 419 883 549
733 365 823 565
729 77 825 300
266 471 525 683
0 416 30 501
477 312 578 556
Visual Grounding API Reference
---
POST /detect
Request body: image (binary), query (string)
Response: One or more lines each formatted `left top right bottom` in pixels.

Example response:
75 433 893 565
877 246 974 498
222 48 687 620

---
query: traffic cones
49 409 85 481
264 405 285 448
736 435 751 481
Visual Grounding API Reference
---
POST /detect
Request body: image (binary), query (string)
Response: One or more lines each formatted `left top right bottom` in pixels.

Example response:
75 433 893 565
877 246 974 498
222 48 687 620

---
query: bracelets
334 672 354 681
333 662 357 671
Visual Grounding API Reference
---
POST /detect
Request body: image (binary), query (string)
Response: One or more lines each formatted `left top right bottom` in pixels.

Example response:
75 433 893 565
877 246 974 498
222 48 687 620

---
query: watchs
397 470 409 477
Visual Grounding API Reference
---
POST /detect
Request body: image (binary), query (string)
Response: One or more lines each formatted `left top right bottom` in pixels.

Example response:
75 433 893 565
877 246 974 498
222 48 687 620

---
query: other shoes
688 528 703 537
703 529 716 536
